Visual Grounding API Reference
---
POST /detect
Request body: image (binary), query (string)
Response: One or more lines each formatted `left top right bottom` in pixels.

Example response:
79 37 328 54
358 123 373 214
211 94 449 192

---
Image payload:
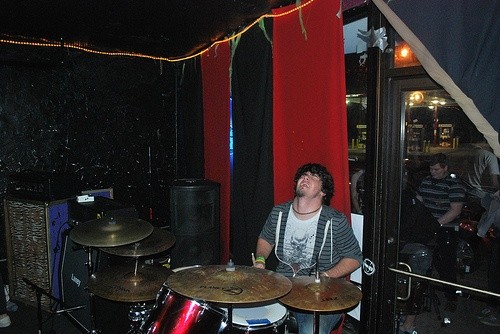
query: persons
460 142 500 207
352 150 471 334
252 163 362 334
0 273 12 328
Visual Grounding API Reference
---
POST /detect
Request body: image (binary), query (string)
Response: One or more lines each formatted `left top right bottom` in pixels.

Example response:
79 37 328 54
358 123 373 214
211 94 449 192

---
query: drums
220 302 291 334
146 292 227 334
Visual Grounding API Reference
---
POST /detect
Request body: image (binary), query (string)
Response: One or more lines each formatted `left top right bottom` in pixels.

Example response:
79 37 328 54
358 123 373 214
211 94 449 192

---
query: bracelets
323 271 329 277
256 255 266 265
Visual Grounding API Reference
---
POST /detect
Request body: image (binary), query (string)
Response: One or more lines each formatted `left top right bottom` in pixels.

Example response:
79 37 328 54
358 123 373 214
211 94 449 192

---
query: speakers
167 179 222 266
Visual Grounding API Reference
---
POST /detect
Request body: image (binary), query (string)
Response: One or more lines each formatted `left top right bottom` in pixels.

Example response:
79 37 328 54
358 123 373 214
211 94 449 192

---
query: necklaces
292 207 321 215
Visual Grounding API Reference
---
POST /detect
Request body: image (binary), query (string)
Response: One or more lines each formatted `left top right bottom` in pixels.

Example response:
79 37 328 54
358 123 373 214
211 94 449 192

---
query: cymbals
87 259 175 302
166 264 293 303
277 276 362 312
69 216 154 247
98 219 176 257
